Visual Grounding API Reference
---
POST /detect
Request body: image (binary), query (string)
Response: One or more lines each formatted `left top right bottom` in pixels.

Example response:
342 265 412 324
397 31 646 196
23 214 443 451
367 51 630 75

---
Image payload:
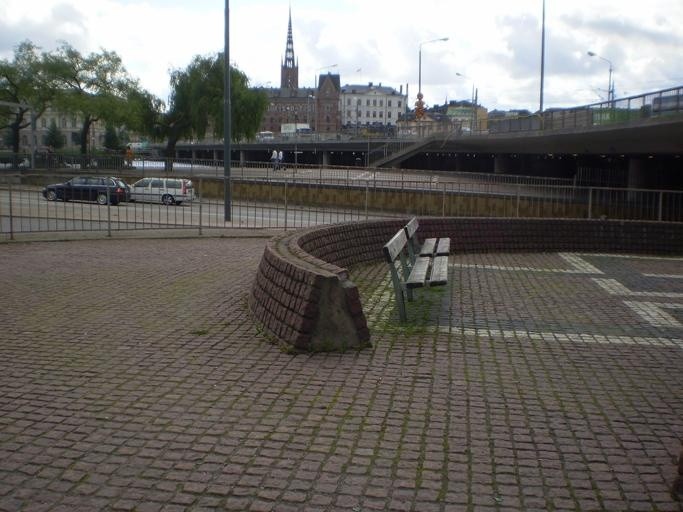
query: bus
255 130 273 142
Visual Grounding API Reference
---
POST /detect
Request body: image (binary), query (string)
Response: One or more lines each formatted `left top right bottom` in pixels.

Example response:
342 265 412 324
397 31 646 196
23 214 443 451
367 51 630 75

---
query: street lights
306 94 314 129
587 50 611 106
314 64 337 94
356 103 360 136
419 37 448 113
456 73 474 134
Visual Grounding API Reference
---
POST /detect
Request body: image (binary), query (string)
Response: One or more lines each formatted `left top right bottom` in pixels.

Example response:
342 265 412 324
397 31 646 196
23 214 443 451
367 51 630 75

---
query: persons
276 147 286 171
126 146 133 169
269 147 277 170
46 148 52 167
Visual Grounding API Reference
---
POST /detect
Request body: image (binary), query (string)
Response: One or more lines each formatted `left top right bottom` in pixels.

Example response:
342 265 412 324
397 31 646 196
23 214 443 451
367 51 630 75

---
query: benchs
404 216 451 264
383 227 448 320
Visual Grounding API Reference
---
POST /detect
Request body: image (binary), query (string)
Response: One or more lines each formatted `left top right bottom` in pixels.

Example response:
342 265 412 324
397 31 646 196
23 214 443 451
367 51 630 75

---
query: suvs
0 156 30 169
41 175 131 208
125 176 196 207
